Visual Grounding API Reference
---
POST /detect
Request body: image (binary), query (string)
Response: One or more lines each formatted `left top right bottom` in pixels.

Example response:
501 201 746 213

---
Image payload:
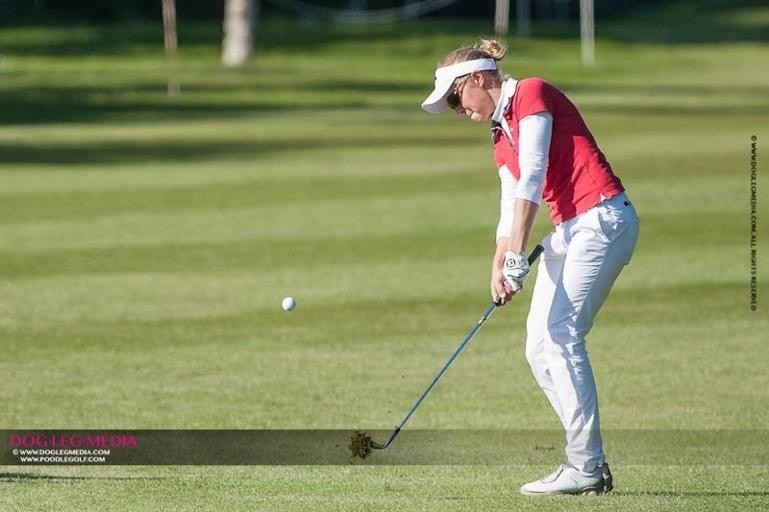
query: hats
418 58 498 114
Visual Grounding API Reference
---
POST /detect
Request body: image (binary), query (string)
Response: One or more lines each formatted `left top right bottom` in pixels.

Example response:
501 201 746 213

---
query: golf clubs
351 245 544 449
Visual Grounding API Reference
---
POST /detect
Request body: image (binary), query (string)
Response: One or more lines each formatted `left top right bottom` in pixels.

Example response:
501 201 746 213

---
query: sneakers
602 459 616 492
520 463 606 500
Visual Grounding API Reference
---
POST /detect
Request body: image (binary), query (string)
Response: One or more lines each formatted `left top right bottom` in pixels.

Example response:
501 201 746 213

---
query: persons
418 35 640 497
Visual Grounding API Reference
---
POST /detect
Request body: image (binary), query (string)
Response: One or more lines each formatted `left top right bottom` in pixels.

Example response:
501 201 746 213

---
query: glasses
445 77 467 111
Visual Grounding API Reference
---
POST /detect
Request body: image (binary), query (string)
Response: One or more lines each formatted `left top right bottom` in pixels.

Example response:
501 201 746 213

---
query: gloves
503 247 530 293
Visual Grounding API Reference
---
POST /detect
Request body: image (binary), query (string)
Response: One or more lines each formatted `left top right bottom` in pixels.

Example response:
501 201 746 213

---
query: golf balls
281 296 295 312
